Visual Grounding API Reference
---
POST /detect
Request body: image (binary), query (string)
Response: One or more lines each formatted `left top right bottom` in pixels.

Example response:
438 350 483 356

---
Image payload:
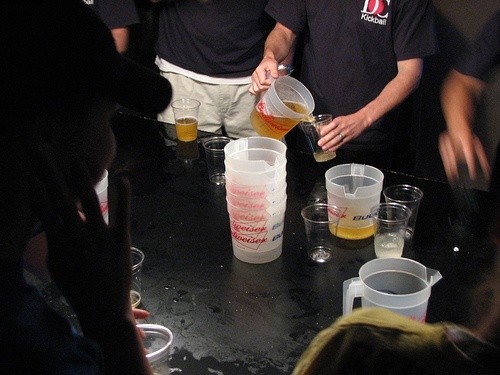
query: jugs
78 169 112 227
223 138 287 264
341 258 443 324
250 71 315 141
324 163 384 240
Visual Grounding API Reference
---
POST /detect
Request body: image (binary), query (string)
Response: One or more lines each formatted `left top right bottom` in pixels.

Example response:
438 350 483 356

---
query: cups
128 248 144 309
382 184 417 240
369 203 412 258
171 98 201 142
298 114 336 163
134 324 172 375
301 204 339 262
202 136 230 184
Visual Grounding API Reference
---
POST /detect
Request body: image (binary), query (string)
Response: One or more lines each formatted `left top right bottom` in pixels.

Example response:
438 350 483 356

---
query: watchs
278 64 295 75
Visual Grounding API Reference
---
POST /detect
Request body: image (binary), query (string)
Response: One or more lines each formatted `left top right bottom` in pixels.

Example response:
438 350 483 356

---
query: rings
338 134 344 139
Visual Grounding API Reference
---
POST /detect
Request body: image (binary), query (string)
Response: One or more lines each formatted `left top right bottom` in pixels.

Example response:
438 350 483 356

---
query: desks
36 111 500 375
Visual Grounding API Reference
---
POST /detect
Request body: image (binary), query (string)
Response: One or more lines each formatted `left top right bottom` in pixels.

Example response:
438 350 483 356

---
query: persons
81 0 141 55
289 139 500 375
0 0 160 374
437 9 500 194
154 0 296 149
248 0 441 176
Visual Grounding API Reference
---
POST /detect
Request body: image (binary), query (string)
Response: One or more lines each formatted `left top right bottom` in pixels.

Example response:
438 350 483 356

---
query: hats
0 0 171 132
